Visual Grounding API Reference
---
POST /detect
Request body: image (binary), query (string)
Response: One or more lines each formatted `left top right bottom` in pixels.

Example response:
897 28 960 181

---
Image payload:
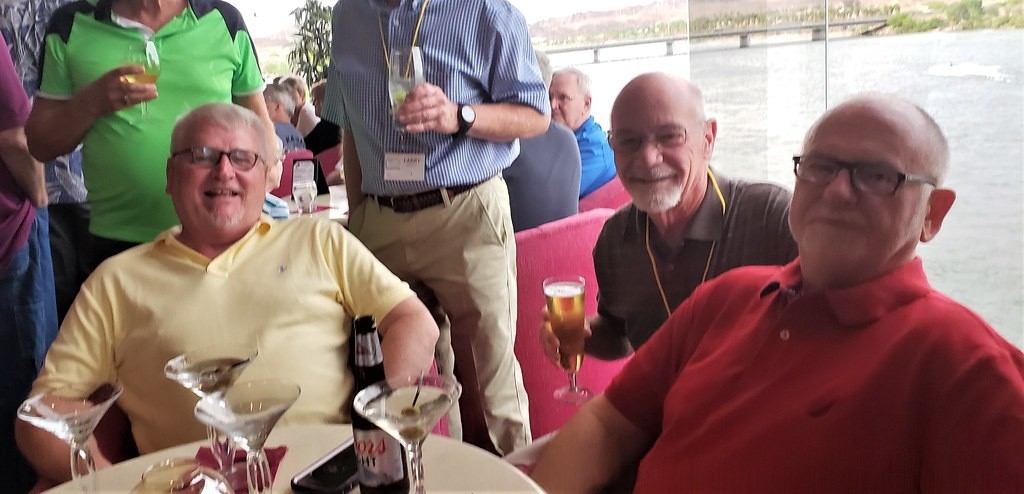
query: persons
540 71 799 369
318 0 553 459
528 91 1024 494
0 0 90 494
24 0 276 274
261 74 343 219
15 101 441 483
502 49 617 233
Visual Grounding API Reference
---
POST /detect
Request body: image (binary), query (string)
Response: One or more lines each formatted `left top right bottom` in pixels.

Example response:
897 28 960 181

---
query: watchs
452 103 477 138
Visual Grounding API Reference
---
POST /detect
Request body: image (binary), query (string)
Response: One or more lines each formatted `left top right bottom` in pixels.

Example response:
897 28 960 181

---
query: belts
364 177 489 213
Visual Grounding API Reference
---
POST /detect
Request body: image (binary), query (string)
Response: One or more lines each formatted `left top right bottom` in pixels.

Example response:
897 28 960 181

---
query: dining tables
35 423 551 494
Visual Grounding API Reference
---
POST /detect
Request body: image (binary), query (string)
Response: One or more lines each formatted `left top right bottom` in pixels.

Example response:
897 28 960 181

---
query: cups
294 179 317 217
389 46 425 131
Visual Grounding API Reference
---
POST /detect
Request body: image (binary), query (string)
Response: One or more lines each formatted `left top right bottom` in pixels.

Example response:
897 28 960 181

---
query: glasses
172 146 265 172
607 125 687 149
792 152 937 199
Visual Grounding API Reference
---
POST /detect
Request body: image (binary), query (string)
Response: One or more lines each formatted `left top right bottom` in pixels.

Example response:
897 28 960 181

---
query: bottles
354 315 410 494
128 456 236 494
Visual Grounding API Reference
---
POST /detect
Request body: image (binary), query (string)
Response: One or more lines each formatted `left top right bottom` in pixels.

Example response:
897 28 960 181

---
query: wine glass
194 380 301 494
15 378 124 494
542 275 594 407
125 41 166 125
164 345 264 490
353 374 462 494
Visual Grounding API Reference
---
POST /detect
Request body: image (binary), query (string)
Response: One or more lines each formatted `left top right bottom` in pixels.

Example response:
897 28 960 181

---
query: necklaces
376 0 429 77
646 167 725 317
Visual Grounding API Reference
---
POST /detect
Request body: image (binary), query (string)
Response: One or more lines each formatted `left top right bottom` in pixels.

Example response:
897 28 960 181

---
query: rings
121 93 131 107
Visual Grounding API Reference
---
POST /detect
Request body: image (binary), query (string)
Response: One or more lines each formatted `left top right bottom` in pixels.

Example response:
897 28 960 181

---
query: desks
280 183 348 226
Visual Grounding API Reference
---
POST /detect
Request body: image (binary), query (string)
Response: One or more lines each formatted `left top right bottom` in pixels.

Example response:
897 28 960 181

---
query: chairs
513 207 638 472
270 150 314 198
315 142 342 186
